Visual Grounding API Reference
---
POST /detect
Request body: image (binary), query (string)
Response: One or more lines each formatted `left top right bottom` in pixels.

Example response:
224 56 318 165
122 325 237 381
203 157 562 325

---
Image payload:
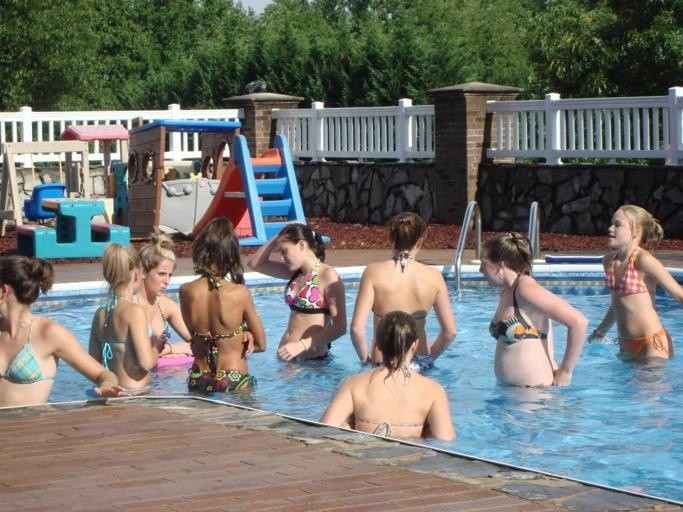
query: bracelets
593 327 605 339
299 337 309 353
168 342 173 354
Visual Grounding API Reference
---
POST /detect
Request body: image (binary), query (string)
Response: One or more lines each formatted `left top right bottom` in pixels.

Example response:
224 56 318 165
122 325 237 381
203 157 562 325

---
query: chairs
24 183 66 220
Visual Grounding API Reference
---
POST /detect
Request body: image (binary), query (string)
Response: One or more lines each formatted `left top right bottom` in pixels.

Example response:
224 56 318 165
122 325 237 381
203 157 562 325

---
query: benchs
17 220 130 257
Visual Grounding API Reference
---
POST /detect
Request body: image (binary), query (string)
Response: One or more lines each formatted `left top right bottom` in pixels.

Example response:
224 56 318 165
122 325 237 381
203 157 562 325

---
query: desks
42 197 105 258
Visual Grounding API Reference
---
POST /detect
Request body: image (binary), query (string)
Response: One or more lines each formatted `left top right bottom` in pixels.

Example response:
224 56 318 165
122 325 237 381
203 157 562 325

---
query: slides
193 148 282 237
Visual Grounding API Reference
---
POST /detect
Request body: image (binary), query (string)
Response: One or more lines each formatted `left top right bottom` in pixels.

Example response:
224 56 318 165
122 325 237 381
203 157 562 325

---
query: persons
478 231 588 389
178 215 267 395
351 212 457 372
249 219 346 363
317 310 456 442
88 242 167 390
133 244 194 368
588 204 683 359
0 254 132 408
